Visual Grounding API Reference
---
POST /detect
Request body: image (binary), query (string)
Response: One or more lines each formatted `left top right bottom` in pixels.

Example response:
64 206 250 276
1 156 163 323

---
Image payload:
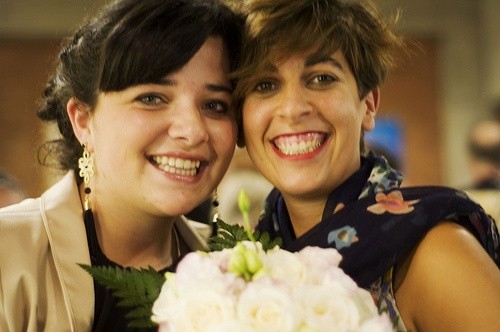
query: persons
0 0 239 332
221 0 500 332
446 119 500 190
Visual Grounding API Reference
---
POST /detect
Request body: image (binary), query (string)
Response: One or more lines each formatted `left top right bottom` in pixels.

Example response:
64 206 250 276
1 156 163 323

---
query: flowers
75 188 396 332
